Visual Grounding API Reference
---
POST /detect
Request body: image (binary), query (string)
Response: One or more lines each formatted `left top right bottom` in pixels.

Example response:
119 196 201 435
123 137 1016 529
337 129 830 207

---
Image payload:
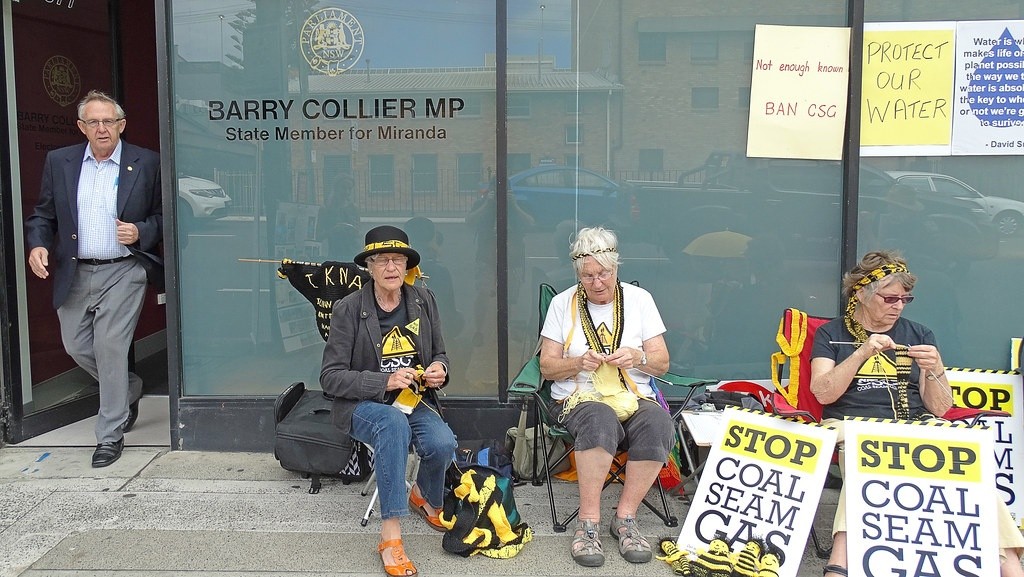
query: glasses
582 273 611 284
370 257 408 264
877 294 914 303
80 118 125 126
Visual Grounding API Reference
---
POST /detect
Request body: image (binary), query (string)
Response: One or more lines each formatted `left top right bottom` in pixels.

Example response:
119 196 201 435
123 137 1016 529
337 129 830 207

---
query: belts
76 255 135 266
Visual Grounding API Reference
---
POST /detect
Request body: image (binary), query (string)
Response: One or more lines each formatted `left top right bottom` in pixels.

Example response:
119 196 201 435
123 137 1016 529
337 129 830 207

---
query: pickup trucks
622 152 1000 294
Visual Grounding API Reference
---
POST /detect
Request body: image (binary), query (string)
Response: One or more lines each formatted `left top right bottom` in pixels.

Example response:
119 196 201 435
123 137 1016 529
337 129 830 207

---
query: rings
405 372 410 379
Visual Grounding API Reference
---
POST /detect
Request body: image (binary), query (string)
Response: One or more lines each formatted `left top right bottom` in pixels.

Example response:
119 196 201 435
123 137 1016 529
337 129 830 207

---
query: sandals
409 484 448 532
610 512 652 563
571 515 605 567
378 537 417 576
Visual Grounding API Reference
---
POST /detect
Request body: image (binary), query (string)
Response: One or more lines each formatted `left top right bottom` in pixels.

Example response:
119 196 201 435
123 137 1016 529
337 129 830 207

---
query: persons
319 226 457 577
27 90 161 468
318 175 363 262
407 216 463 338
519 218 584 330
467 176 536 346
709 237 801 364
810 252 1024 577
539 228 676 565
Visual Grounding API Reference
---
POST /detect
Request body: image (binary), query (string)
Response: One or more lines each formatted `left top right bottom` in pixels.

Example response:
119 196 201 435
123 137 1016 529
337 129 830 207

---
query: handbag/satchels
505 423 571 479
442 469 532 559
274 382 376 494
454 439 512 475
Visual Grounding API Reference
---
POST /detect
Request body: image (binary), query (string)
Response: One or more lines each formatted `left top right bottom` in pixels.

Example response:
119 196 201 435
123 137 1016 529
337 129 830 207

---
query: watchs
638 351 647 368
925 370 944 381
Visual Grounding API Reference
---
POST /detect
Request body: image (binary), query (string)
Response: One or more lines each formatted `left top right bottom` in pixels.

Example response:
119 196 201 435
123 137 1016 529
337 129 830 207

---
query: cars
469 165 651 236
177 171 236 227
866 165 1024 242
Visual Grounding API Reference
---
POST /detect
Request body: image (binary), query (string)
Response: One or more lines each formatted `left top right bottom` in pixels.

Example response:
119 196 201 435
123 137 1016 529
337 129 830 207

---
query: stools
358 442 419 526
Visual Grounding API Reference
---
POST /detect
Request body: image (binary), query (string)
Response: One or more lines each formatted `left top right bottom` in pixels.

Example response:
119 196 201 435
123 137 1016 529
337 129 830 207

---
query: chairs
506 280 720 532
771 309 1011 555
669 390 764 497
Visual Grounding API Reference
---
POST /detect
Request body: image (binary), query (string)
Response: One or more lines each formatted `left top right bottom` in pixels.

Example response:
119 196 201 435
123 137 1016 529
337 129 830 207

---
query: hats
878 184 925 212
404 217 444 257
333 173 355 196
354 226 421 270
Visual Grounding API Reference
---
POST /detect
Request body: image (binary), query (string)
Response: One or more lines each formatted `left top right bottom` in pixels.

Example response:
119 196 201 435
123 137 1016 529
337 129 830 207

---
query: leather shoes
120 393 143 432
92 436 124 467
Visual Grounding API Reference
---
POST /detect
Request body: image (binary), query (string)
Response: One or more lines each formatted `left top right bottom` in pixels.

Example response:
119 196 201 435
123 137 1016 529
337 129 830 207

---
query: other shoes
472 331 484 347
821 562 849 575
